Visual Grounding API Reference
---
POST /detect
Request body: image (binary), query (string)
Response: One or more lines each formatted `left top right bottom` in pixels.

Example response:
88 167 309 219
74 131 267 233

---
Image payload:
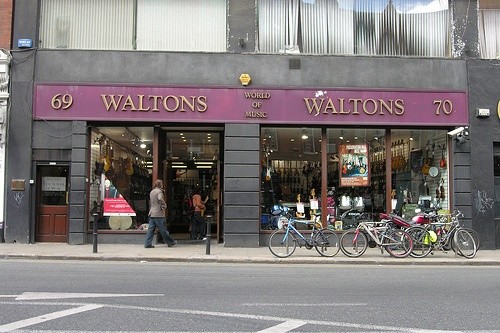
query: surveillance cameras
447 127 464 137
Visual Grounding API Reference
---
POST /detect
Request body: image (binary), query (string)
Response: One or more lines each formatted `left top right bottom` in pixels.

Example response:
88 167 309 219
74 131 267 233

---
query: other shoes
200 237 207 239
194 237 199 240
145 244 154 248
168 240 177 247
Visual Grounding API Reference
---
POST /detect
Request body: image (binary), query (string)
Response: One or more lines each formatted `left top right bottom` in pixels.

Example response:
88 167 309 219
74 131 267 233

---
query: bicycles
340 207 481 259
268 212 340 258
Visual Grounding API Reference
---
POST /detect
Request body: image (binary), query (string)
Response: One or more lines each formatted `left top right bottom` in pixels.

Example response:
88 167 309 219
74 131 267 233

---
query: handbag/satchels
188 205 195 216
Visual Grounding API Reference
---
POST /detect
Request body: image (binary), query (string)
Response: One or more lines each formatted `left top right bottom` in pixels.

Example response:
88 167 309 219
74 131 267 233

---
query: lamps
239 38 244 46
474 108 492 117
301 129 309 140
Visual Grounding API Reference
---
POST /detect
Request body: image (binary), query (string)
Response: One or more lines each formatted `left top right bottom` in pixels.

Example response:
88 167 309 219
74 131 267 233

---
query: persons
143 180 177 248
191 187 207 241
